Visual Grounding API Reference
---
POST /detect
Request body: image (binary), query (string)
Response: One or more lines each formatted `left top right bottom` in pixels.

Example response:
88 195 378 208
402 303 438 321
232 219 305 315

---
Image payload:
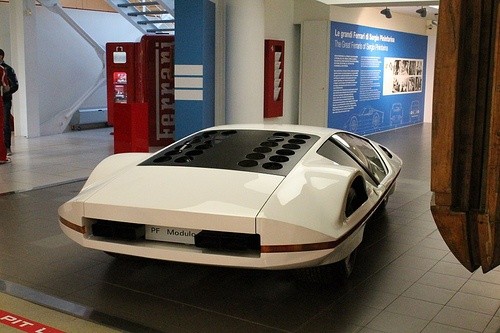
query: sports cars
56 123 403 279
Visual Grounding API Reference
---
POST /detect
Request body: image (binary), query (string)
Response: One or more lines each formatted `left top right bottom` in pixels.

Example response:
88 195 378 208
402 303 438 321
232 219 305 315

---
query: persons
0 48 20 157
0 64 11 164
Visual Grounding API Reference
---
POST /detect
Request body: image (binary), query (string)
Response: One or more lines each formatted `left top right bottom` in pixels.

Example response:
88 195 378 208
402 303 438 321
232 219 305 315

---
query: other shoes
0 158 10 164
7 148 12 156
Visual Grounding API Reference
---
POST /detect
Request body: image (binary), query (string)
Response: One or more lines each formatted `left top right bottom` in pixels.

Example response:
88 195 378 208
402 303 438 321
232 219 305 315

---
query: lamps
380 4 391 19
415 4 427 18
